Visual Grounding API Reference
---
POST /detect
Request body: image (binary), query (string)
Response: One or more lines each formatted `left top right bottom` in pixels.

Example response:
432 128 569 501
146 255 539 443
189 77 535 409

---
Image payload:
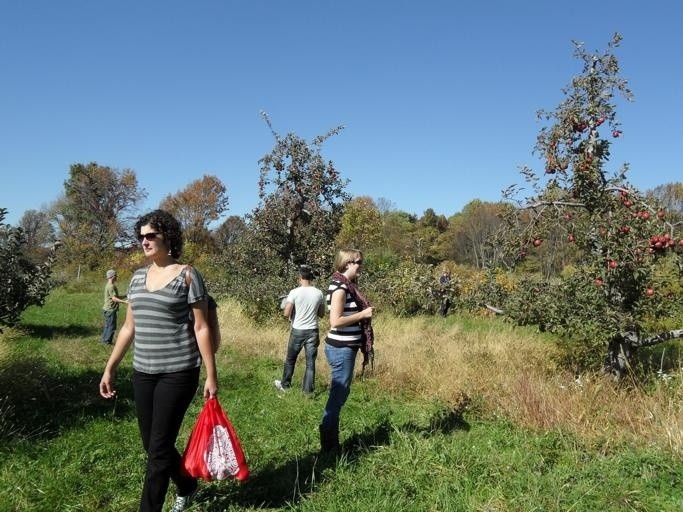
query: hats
107 270 116 278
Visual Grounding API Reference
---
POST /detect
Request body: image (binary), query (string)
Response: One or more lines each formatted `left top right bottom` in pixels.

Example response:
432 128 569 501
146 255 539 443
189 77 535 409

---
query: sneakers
274 379 289 391
174 484 198 512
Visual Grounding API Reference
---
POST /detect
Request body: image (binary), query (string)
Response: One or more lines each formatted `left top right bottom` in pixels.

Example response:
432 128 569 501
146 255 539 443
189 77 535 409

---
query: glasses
347 260 362 265
138 233 164 241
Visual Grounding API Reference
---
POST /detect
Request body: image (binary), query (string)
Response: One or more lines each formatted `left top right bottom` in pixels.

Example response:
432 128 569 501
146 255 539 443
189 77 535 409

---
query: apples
521 116 683 296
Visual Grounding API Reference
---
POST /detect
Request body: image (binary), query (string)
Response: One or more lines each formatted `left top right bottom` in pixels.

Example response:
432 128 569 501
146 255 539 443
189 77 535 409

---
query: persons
101 270 129 344
274 267 326 399
319 249 373 461
99 211 220 512
440 269 452 317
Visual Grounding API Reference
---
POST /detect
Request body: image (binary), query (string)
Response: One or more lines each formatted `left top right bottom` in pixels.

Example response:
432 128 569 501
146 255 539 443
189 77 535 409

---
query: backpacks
185 264 220 352
